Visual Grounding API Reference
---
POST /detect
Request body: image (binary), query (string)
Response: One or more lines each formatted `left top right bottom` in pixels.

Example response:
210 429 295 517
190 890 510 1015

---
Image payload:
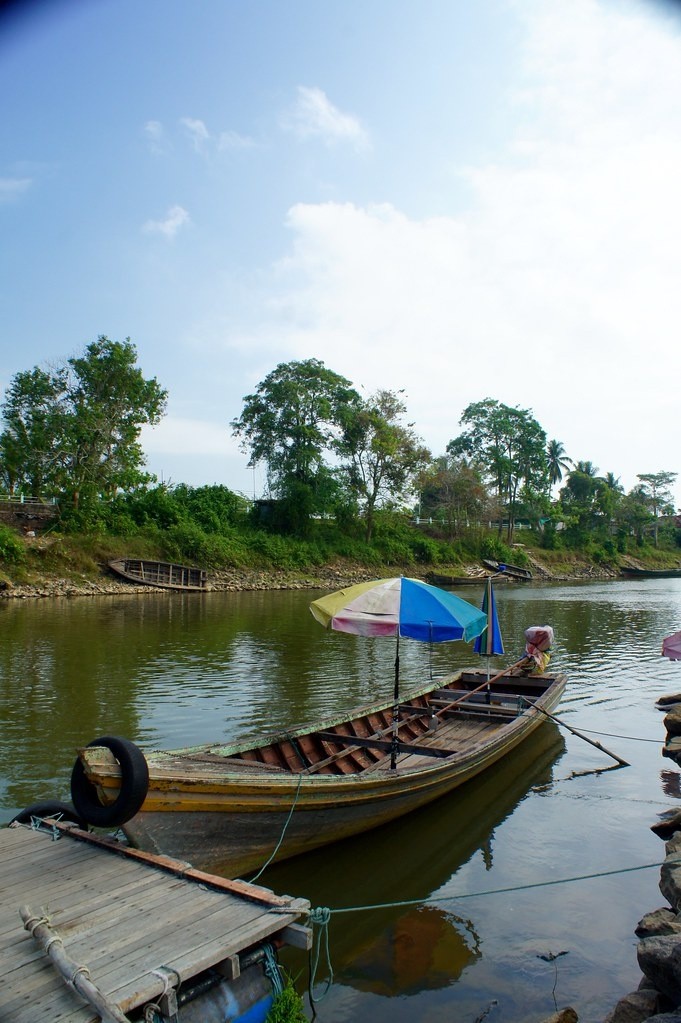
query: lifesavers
71 736 150 827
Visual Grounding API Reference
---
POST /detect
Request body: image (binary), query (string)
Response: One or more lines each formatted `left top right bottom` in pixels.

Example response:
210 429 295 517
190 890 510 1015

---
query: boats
72 666 570 886
619 566 681 578
424 571 509 584
97 558 209 590
481 558 533 582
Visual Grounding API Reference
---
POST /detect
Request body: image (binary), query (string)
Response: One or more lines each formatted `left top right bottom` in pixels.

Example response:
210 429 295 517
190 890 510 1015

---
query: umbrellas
474 578 505 692
309 578 488 770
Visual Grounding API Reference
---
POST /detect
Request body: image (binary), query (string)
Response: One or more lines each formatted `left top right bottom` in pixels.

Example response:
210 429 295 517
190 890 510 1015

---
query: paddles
519 696 631 766
